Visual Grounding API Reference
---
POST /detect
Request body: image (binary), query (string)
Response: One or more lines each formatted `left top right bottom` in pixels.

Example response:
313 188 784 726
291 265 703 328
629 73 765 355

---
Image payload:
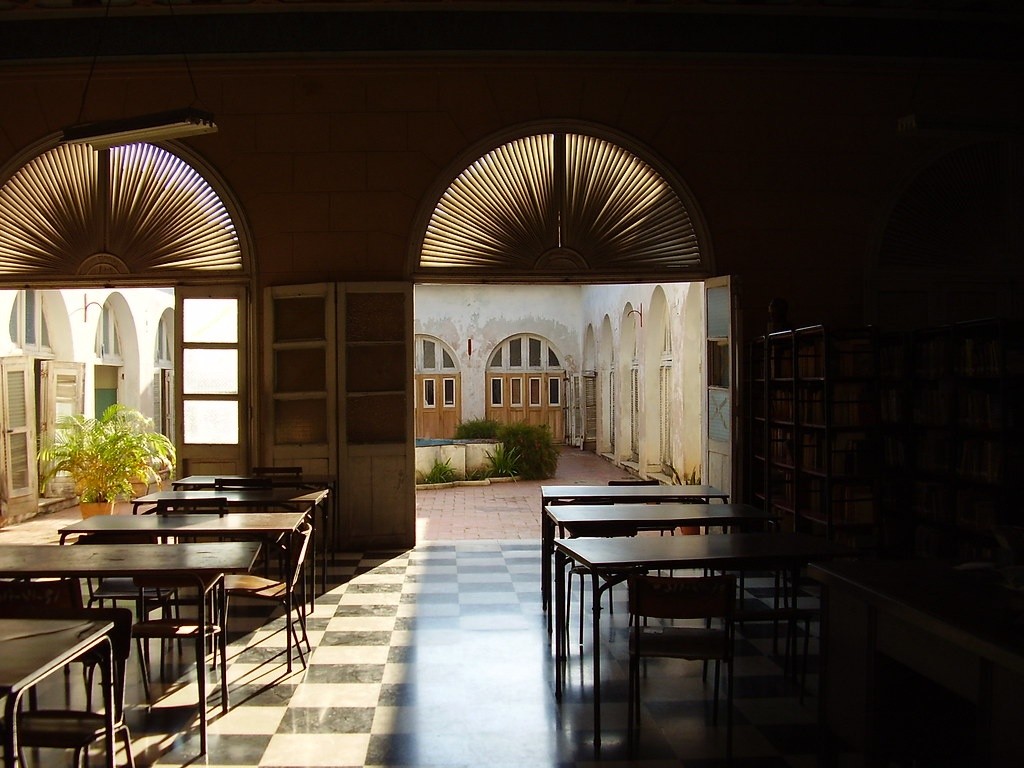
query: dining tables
58 513 305 673
130 489 330 612
0 619 116 768
541 485 730 610
0 542 262 754
172 475 337 592
544 503 775 663
553 531 828 751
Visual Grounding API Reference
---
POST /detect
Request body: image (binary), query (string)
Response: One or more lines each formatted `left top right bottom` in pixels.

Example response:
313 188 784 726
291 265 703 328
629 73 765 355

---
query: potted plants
36 403 176 519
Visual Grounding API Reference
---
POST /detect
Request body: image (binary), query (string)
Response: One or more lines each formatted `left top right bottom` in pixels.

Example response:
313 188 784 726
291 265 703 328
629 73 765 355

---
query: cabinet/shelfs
745 323 1024 550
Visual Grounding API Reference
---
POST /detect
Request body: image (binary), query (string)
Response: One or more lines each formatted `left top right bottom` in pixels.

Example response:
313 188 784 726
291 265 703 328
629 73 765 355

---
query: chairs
215 479 267 490
627 574 736 758
209 523 312 669
253 467 303 475
0 580 82 618
0 607 136 768
131 586 221 699
157 497 229 516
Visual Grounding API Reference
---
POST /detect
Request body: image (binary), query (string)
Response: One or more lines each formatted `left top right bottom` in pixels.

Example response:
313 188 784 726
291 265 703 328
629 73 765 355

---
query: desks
808 557 1024 768
